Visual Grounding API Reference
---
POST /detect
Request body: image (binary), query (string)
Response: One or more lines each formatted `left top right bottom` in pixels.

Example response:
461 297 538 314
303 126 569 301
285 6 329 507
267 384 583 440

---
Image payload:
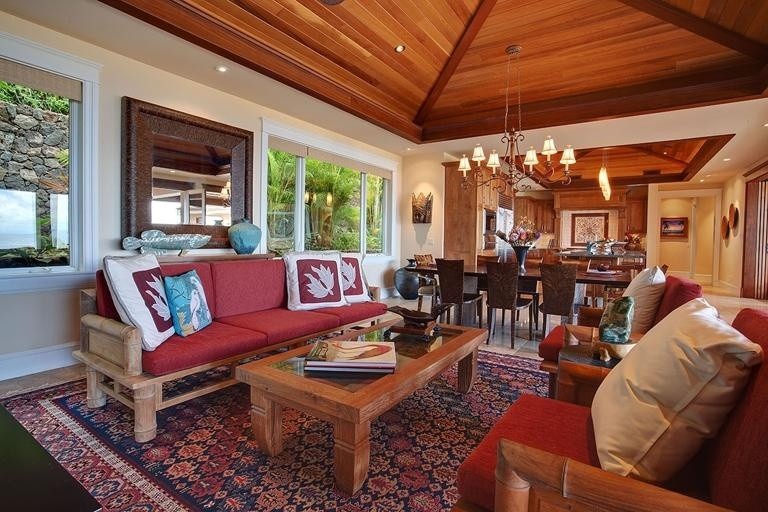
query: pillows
621 265 666 334
591 296 766 483
96 253 371 353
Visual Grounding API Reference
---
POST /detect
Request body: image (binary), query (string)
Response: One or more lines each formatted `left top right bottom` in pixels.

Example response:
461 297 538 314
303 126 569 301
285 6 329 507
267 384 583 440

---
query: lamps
458 45 577 199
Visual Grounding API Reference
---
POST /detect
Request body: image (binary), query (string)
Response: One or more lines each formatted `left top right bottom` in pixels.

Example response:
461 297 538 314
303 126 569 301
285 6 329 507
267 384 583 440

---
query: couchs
70 255 389 443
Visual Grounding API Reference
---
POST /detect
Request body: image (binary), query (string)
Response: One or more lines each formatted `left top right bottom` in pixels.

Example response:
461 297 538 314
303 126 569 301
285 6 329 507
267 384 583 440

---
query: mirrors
120 96 254 250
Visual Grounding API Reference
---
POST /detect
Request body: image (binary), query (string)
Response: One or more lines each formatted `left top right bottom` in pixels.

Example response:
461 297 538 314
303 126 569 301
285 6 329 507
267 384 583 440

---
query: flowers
510 215 541 246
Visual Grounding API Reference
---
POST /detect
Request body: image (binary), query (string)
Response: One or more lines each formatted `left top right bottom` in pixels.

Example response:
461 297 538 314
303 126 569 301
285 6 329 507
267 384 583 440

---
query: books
305 339 396 377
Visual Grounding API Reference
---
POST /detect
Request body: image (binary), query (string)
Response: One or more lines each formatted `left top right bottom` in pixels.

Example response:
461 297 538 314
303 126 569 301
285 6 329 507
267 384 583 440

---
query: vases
393 259 420 299
512 246 530 275
228 220 262 255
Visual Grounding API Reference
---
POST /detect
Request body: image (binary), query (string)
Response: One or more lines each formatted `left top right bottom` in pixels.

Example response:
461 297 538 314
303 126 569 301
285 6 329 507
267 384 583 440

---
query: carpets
0 346 550 512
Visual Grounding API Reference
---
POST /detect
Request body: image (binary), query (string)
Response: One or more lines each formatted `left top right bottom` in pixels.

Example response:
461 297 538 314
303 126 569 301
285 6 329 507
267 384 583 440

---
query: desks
236 319 489 496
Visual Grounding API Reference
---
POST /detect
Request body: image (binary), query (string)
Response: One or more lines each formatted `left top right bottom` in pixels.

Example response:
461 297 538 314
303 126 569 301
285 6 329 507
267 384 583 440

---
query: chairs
539 275 703 399
448 307 767 512
415 252 669 349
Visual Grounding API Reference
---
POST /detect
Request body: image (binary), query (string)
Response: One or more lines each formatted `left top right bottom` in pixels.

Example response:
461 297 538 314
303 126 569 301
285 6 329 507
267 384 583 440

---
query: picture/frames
660 217 688 240
571 212 609 245
